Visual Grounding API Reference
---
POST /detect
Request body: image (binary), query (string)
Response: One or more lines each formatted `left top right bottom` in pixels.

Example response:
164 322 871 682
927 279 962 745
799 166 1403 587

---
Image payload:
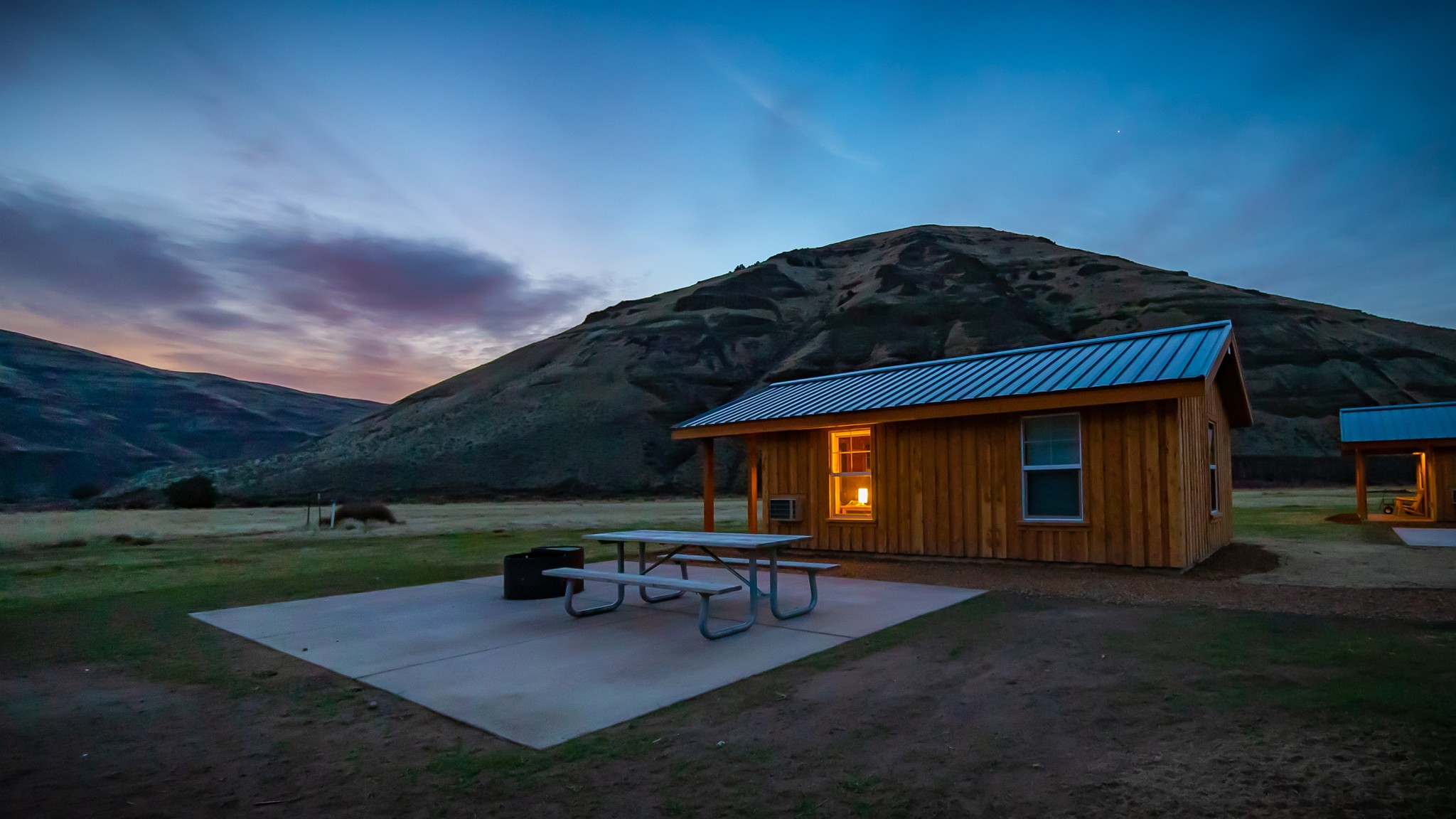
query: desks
565 530 816 640
840 505 872 514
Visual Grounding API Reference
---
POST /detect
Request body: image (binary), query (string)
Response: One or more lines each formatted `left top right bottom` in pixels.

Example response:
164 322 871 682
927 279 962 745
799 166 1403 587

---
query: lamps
858 488 868 506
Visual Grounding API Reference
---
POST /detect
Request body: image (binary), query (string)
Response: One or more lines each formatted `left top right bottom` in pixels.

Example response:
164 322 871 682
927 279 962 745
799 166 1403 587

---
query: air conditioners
769 499 804 521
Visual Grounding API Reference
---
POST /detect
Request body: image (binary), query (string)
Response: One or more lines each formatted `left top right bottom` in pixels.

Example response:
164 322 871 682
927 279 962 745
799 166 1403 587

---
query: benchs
543 554 841 640
